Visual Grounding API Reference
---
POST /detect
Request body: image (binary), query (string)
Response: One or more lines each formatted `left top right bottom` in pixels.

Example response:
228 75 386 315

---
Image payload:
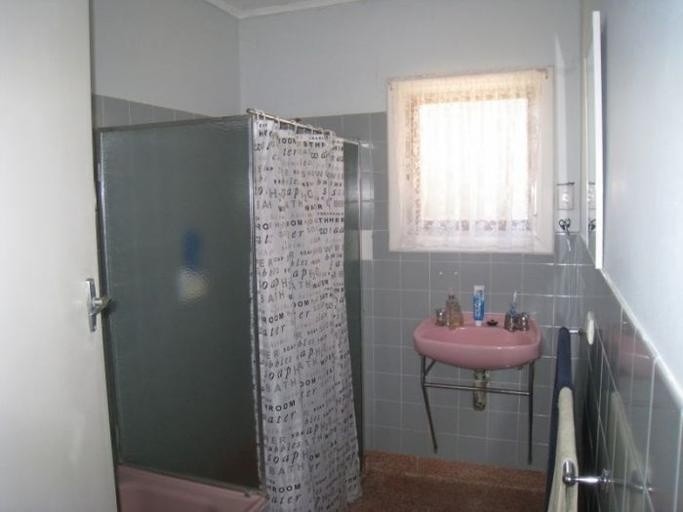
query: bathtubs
115 464 264 512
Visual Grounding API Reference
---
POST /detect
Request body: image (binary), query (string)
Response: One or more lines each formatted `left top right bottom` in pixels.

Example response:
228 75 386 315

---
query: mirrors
582 10 605 269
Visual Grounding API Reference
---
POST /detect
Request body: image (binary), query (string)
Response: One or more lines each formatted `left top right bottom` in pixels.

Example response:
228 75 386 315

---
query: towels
546 327 582 511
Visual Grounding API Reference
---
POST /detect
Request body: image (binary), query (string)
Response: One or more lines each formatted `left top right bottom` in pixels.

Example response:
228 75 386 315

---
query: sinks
414 312 542 370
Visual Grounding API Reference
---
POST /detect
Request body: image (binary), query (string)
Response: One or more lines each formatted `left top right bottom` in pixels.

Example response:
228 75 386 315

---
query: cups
431 307 447 327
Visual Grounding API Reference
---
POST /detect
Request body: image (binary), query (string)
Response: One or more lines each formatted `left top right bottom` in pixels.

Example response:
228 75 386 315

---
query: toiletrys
445 292 459 327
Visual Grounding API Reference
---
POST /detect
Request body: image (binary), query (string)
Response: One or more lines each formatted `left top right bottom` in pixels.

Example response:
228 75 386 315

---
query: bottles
446 295 458 330
